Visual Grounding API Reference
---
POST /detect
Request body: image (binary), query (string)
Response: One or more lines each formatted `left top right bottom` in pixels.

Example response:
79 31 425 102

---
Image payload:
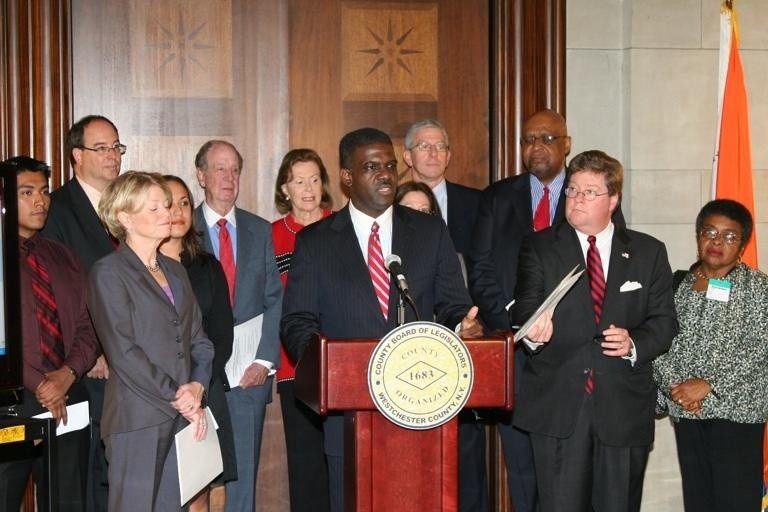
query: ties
217 219 237 306
533 186 550 231
21 239 65 369
368 222 390 322
584 234 607 396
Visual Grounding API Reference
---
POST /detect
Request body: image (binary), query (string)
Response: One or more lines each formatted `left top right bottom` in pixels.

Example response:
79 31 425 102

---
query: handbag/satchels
654 386 670 420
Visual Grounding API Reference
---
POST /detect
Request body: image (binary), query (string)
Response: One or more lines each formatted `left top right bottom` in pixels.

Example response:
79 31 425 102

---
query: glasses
418 209 434 215
700 224 741 244
565 187 608 201
83 144 126 155
520 135 567 145
410 142 448 152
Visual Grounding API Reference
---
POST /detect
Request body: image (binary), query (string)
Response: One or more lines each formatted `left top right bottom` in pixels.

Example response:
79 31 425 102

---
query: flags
712 4 768 509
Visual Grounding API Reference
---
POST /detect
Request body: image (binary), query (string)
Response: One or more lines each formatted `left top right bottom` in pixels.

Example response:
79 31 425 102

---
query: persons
1 109 768 512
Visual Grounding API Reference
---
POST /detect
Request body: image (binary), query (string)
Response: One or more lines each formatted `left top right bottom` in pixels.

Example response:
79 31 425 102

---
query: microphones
382 255 413 296
